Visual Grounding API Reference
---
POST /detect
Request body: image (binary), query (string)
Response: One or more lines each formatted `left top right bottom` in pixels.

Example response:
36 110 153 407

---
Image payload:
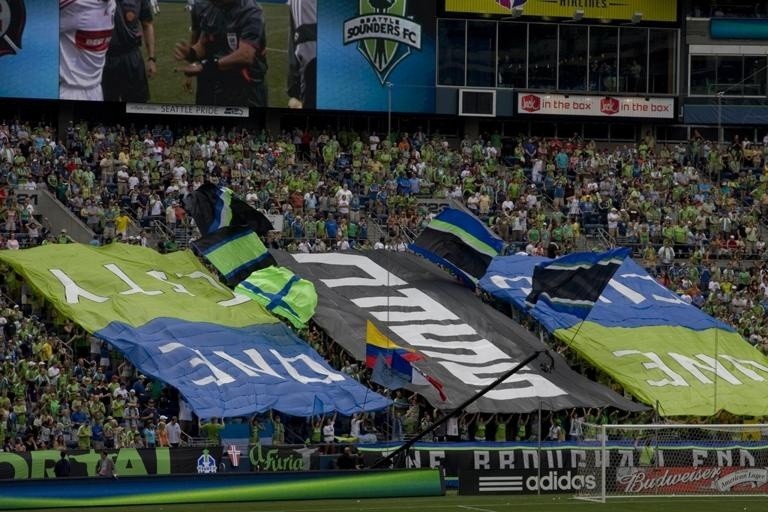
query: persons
0 120 768 452
52 449 71 477
630 60 642 93
94 450 119 479
59 0 316 110
335 445 359 469
634 435 654 468
